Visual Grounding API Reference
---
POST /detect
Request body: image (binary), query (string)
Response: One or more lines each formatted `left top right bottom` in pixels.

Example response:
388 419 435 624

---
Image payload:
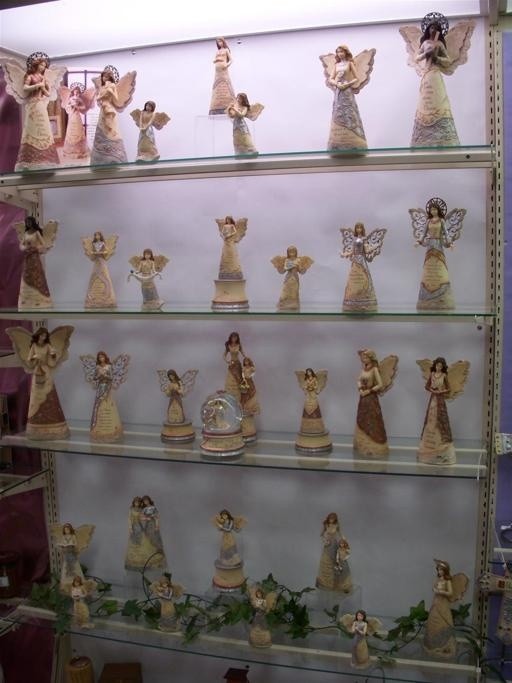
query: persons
60 575 99 629
81 230 119 307
129 99 171 164
224 92 265 160
124 496 164 571
397 11 475 153
208 35 236 115
148 572 186 631
214 215 249 279
408 197 467 310
247 585 278 649
213 399 227 428
319 44 377 157
293 366 330 434
315 512 345 591
334 539 353 593
415 356 471 465
353 347 399 455
5 324 76 439
1 51 67 179
78 351 131 442
12 215 59 309
270 244 314 310
138 494 164 555
223 332 246 404
90 63 137 173
156 368 200 423
422 558 469 658
210 508 249 567
47 521 97 591
57 80 98 162
338 609 383 670
339 221 387 312
238 357 261 415
127 247 170 309
